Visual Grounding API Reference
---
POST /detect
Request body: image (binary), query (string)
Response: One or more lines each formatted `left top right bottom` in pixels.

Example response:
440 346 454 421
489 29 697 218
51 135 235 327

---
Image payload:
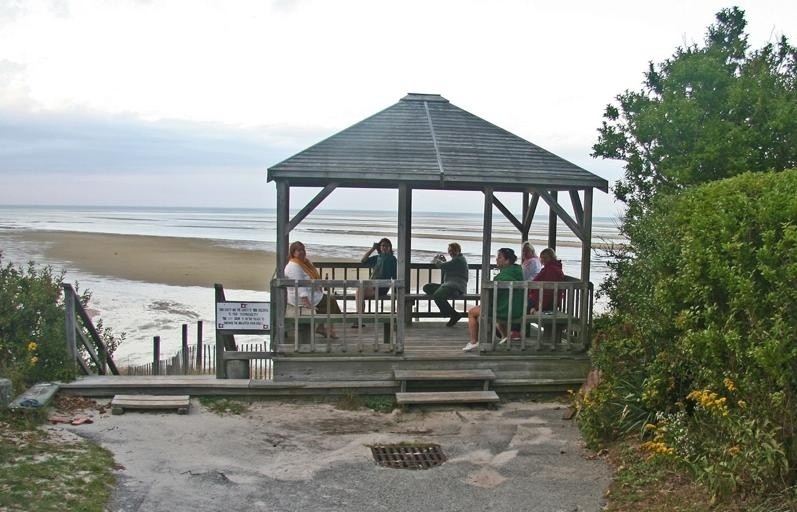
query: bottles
324 273 329 291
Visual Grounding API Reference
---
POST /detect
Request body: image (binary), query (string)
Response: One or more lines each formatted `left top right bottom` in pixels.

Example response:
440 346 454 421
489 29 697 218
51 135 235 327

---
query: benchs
476 310 579 349
282 304 397 350
324 293 482 322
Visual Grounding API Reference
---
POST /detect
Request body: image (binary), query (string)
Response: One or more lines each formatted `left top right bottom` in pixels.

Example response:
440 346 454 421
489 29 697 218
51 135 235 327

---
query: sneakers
462 341 479 352
315 324 340 340
446 313 462 327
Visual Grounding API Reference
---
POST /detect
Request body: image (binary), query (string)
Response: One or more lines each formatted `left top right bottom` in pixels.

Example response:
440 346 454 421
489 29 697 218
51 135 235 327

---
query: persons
283 240 342 341
460 248 527 352
421 243 468 327
519 239 542 290
509 247 565 341
349 238 398 329
